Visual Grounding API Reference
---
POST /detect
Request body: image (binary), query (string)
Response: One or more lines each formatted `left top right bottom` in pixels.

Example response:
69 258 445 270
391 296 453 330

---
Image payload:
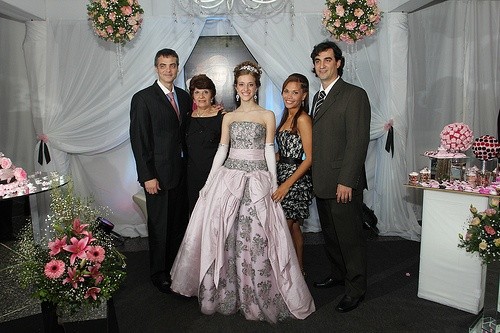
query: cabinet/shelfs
404 152 500 316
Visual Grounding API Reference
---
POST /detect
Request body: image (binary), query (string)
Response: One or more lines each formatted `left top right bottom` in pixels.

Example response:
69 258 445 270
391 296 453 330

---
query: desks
0 171 71 242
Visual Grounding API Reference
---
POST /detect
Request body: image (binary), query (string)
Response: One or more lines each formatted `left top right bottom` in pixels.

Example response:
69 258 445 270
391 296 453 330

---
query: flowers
457 197 500 264
320 0 384 44
6 175 125 312
0 152 28 197
87 0 144 43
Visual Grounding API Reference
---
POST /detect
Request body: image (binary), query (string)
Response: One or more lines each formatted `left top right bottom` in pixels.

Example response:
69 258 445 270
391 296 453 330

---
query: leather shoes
314 276 347 287
335 293 358 312
156 277 172 293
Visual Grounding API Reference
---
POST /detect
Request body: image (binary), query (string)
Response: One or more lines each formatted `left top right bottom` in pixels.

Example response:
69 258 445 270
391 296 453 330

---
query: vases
57 296 108 323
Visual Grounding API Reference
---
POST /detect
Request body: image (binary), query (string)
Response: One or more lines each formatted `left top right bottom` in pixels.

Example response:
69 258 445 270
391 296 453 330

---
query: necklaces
197 110 206 117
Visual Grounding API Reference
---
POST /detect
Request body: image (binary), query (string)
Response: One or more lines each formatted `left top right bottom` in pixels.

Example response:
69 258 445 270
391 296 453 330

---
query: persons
271 71 312 277
309 40 372 313
131 49 231 292
181 74 229 219
169 61 315 325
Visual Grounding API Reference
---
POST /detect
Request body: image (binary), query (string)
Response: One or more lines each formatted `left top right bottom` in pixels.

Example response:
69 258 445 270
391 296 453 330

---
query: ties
168 92 179 118
313 91 326 119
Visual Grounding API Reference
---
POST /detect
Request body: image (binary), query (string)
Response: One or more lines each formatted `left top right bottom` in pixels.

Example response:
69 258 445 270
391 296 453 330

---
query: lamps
172 0 296 35
